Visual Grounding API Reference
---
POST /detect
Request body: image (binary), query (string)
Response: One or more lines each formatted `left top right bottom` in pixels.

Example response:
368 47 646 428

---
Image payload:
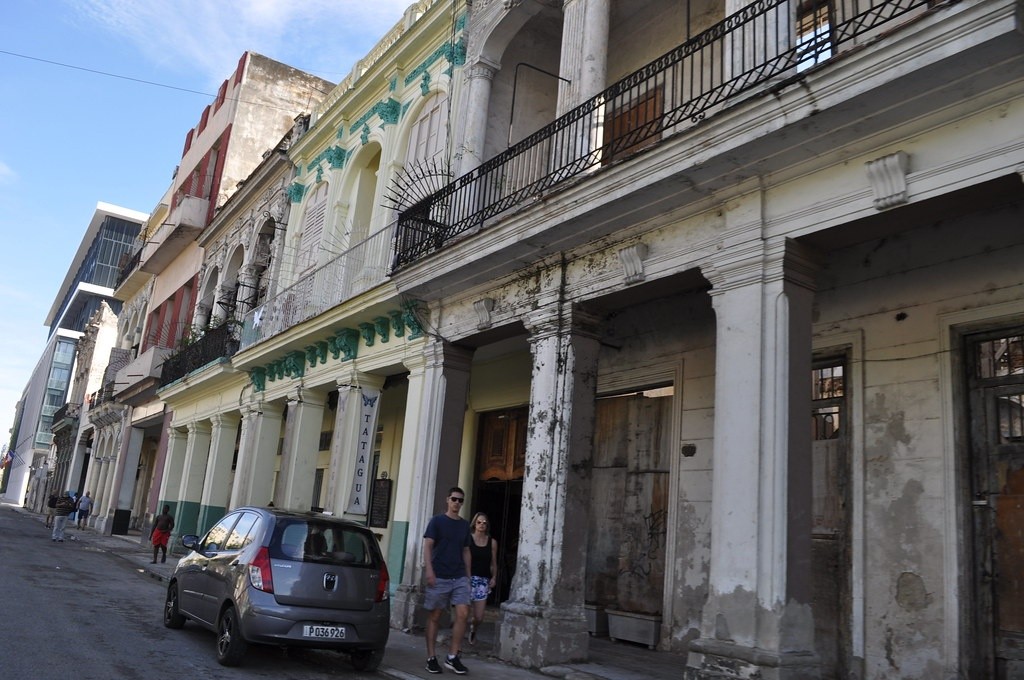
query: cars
162 504 390 673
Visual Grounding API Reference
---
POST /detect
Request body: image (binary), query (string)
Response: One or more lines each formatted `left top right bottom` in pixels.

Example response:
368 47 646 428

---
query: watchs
466 575 472 581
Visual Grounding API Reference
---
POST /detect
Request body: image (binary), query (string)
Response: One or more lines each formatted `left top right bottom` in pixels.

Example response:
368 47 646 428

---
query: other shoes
46 526 50 529
77 527 79 530
53 539 56 541
82 529 84 530
150 562 158 564
161 559 166 563
58 540 63 542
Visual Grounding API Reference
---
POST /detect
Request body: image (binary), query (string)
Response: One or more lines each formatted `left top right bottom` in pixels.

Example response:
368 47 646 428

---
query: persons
148 504 175 565
44 489 93 543
423 487 497 675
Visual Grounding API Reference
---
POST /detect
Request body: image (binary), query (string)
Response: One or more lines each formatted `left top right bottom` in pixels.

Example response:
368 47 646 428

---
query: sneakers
425 657 443 673
468 625 477 645
444 654 469 674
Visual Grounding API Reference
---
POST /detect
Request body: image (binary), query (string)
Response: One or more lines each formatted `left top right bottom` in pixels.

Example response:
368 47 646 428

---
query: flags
0 450 15 470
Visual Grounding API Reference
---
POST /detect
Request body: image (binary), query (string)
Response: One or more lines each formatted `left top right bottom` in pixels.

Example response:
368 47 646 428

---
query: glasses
476 520 487 524
448 497 464 503
